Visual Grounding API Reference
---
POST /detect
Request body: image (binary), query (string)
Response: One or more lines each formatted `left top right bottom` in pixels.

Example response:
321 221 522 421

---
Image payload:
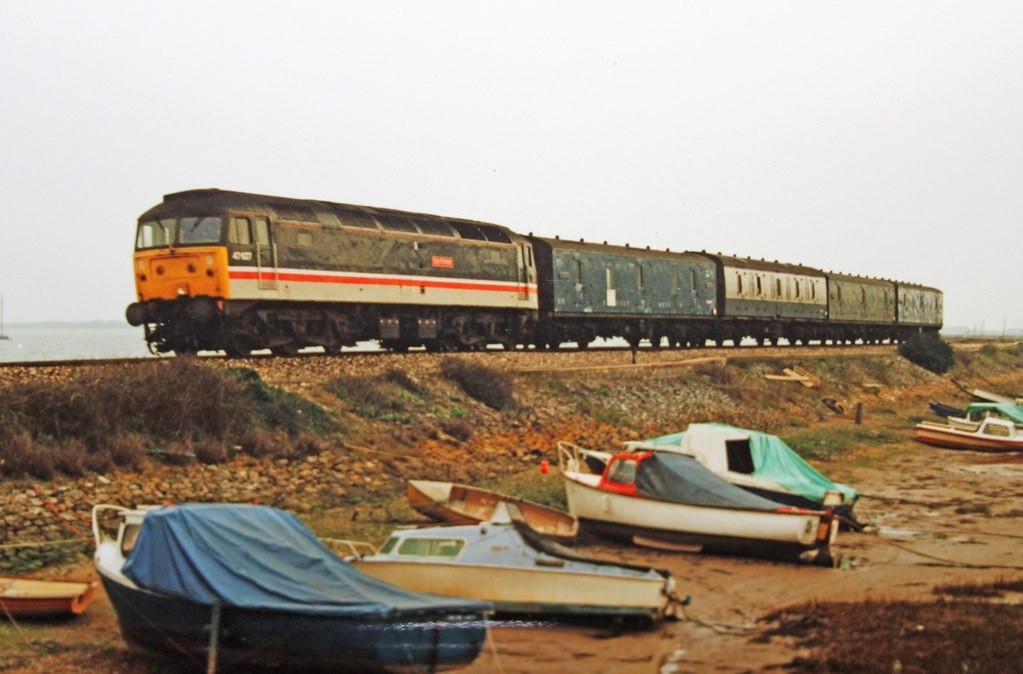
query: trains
124 190 944 359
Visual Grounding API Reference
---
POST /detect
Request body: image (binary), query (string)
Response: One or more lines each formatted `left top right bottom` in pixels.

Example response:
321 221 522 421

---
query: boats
556 421 860 569
316 501 691 629
914 377 1023 451
407 480 579 545
90 502 496 674
0 575 95 621
